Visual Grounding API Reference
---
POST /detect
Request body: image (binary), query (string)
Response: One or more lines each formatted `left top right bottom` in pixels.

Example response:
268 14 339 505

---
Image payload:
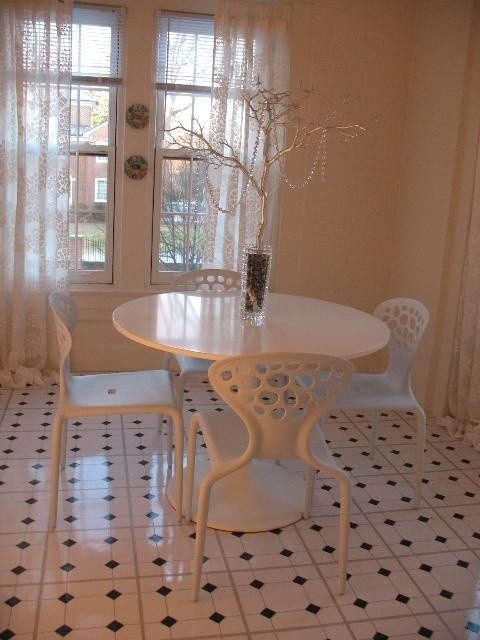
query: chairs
180 350 354 603
45 289 188 535
154 268 247 465
291 295 429 510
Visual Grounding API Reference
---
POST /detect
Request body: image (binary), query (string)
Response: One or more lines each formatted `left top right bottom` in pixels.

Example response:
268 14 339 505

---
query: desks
111 288 392 533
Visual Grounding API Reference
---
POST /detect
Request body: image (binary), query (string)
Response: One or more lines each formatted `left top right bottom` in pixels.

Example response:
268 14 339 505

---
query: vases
239 242 275 328
